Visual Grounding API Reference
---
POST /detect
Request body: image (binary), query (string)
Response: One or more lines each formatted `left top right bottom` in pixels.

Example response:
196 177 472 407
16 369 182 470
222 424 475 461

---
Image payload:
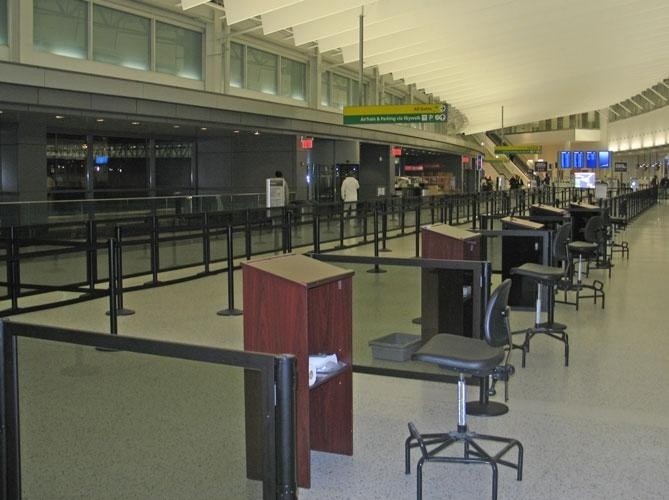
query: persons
651 174 660 192
275 170 290 205
542 172 550 187
660 176 669 195
480 173 494 193
509 175 525 190
336 171 360 229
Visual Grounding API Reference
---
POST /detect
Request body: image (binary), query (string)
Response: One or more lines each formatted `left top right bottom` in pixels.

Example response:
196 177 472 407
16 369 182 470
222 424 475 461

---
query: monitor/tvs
573 172 596 189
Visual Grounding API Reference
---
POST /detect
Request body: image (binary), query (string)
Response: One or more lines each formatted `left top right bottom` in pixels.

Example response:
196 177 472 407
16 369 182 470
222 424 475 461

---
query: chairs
554 216 604 311
506 223 571 368
405 279 523 500
606 199 629 259
